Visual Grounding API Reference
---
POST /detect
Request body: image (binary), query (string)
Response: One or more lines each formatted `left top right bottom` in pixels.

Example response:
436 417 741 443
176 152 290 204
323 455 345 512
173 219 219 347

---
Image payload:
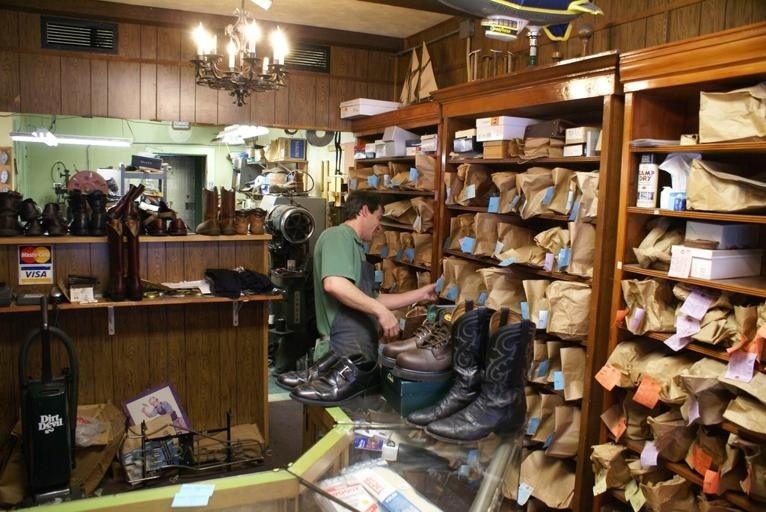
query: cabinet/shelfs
350 18 765 512
0 230 283 478
239 158 309 196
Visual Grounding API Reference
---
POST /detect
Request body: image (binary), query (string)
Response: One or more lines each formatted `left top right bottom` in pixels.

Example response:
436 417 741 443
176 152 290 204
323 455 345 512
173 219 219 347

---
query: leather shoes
288 356 380 406
275 350 366 391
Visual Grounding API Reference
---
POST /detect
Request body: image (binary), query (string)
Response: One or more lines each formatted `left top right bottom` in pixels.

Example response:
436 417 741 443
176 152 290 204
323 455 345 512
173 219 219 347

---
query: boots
0 185 188 237
381 303 536 444
196 187 266 236
103 218 126 302
125 219 142 301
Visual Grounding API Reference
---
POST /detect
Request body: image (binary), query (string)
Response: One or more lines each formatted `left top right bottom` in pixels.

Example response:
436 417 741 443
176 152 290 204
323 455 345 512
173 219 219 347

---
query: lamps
8 115 137 149
189 0 290 109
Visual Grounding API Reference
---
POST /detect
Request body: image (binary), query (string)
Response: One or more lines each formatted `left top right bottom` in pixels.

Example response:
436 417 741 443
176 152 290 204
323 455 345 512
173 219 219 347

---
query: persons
142 397 186 432
311 190 437 366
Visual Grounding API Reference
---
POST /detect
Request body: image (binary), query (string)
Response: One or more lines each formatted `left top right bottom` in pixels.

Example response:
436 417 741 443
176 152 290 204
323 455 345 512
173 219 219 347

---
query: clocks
50 161 66 185
